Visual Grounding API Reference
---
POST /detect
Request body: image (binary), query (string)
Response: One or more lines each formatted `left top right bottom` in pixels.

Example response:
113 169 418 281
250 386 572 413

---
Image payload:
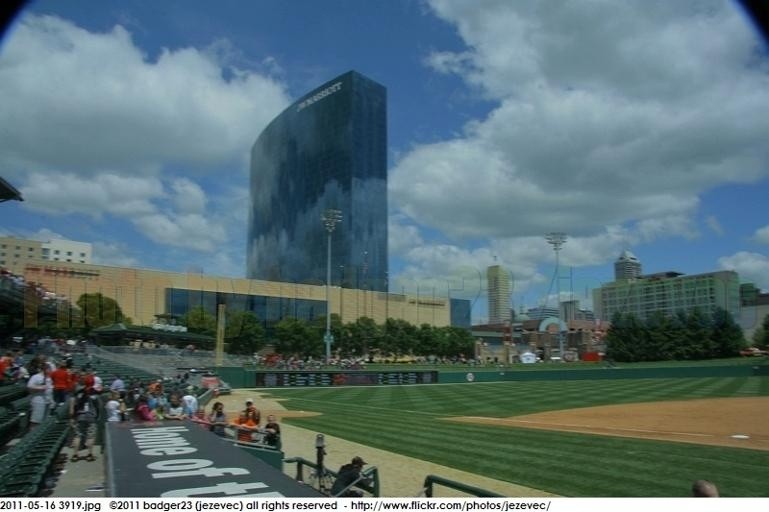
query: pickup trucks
739 347 768 357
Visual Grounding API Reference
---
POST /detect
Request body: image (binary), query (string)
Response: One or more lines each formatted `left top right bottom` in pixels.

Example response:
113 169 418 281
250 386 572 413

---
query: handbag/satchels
77 411 96 423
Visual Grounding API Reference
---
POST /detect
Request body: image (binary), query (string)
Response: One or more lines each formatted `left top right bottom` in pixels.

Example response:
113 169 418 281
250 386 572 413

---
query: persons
330 457 370 497
1 268 498 462
692 480 718 497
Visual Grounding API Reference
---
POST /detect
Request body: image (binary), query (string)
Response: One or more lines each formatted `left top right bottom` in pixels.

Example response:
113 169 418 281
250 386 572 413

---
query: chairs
0 339 257 497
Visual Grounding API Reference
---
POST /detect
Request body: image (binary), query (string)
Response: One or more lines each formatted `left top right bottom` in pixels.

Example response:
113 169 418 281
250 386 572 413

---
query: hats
246 398 253 403
84 376 94 385
352 457 368 464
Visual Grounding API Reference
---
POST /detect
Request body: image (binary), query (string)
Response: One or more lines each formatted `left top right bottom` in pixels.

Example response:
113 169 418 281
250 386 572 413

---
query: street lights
545 231 569 358
321 208 344 364
